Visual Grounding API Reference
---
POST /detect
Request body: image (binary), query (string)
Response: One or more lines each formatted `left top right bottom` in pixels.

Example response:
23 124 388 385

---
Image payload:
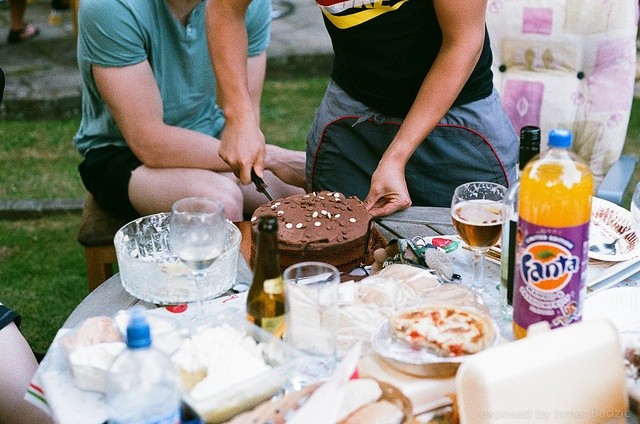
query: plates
588 197 640 262
582 286 640 357
372 301 502 378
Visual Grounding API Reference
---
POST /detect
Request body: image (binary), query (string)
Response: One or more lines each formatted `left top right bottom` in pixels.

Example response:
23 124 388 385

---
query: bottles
501 126 541 319
245 216 290 339
105 314 184 424
512 128 594 340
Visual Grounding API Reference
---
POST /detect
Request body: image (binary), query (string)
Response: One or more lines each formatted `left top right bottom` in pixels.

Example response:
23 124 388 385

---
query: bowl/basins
114 210 242 305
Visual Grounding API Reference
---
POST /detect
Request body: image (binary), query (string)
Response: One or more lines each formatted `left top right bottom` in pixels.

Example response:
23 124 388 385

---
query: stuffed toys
368 242 428 275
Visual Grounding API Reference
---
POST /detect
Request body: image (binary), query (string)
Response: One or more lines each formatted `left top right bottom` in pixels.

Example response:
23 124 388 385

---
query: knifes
251 169 274 202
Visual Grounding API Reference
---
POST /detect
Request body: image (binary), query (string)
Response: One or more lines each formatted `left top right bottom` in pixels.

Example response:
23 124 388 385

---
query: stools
79 188 252 300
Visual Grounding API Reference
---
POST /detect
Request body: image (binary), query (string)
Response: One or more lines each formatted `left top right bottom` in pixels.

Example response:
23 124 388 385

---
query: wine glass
170 196 227 332
450 181 513 304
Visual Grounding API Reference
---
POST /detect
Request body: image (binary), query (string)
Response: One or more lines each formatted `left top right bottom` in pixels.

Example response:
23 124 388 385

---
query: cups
283 262 342 391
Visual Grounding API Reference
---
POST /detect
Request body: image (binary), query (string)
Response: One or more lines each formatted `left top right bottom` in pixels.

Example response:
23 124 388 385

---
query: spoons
590 229 634 255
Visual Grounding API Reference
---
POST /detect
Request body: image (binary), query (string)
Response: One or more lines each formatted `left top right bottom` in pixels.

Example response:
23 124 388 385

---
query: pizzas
391 305 489 354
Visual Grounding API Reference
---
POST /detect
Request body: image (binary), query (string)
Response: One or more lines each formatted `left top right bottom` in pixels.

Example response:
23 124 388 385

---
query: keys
415 237 431 263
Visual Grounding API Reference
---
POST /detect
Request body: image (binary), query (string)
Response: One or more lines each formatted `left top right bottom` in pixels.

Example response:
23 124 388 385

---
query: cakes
247 192 373 276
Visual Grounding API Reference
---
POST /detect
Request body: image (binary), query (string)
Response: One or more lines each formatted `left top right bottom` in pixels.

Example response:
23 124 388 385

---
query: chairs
475 0 637 210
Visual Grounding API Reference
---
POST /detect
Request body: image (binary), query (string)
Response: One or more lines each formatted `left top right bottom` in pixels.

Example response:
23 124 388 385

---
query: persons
0 303 52 424
73 0 306 223
7 1 39 44
205 0 520 217
